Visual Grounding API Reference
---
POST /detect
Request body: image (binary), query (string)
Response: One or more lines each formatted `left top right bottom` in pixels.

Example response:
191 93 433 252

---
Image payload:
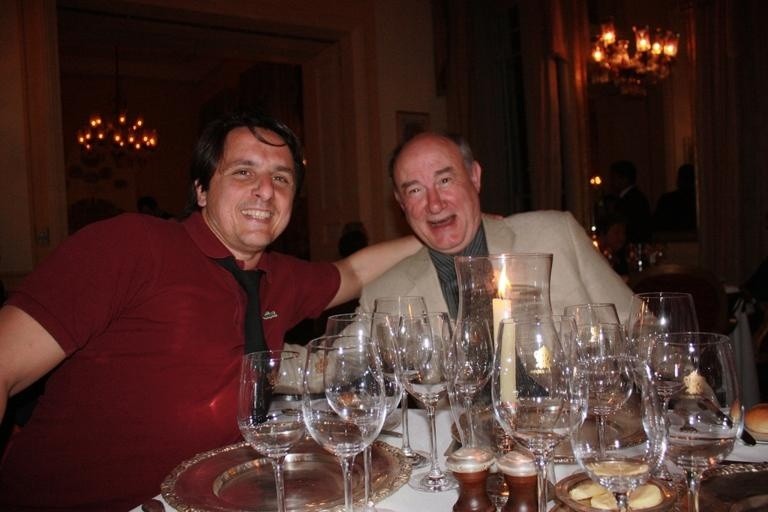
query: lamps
590 0 680 98
73 44 162 160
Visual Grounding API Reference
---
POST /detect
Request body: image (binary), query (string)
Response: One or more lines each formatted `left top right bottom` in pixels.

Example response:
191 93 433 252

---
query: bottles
445 253 561 449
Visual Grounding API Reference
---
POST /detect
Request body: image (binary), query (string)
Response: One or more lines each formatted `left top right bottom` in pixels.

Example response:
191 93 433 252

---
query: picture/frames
396 110 430 231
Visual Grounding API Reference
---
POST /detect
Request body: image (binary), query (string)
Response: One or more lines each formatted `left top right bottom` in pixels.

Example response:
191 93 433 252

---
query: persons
268 127 721 415
648 162 697 236
591 158 652 248
1 103 503 512
593 210 633 289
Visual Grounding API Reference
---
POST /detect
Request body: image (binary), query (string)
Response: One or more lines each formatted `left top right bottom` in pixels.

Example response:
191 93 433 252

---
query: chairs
628 263 730 334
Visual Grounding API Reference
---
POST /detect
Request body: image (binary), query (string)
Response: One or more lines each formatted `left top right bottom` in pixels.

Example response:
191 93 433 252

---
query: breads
743 404 767 441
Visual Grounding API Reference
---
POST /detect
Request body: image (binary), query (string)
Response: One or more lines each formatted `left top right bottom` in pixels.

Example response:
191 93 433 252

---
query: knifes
699 396 756 447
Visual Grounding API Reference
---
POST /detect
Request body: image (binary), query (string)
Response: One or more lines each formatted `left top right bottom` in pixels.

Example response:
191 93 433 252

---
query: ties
218 257 272 420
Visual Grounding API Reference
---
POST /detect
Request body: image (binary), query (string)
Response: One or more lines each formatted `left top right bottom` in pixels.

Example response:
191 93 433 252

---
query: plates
159 435 414 512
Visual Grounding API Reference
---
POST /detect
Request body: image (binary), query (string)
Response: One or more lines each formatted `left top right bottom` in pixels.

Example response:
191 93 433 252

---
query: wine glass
301 289 742 511
237 350 314 511
